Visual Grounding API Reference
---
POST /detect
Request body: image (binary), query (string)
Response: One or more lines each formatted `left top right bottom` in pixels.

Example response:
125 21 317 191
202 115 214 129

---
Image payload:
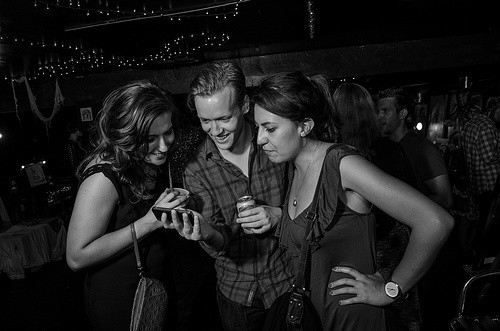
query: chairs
449 268 500 331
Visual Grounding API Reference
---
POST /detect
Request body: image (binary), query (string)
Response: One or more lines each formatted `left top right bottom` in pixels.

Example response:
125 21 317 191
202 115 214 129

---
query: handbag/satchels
129 223 168 331
285 293 305 331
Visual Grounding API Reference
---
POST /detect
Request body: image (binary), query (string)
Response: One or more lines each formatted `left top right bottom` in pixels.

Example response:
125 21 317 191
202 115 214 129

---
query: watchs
383 267 409 304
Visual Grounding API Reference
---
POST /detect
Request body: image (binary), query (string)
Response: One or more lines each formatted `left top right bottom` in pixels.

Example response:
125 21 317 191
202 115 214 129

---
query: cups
165 188 190 208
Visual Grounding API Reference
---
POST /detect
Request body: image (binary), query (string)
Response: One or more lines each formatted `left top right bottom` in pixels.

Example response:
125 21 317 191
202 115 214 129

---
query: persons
65 79 190 331
333 82 500 331
161 60 295 331
253 70 456 331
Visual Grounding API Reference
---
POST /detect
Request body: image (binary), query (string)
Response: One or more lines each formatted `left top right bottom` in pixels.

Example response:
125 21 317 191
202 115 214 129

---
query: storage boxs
0 217 67 279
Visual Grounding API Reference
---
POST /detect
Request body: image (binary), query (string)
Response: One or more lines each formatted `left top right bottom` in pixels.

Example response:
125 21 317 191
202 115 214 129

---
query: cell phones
152 207 195 227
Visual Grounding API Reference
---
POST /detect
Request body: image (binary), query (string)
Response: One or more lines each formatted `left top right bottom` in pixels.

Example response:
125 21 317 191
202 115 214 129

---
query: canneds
236 195 257 234
